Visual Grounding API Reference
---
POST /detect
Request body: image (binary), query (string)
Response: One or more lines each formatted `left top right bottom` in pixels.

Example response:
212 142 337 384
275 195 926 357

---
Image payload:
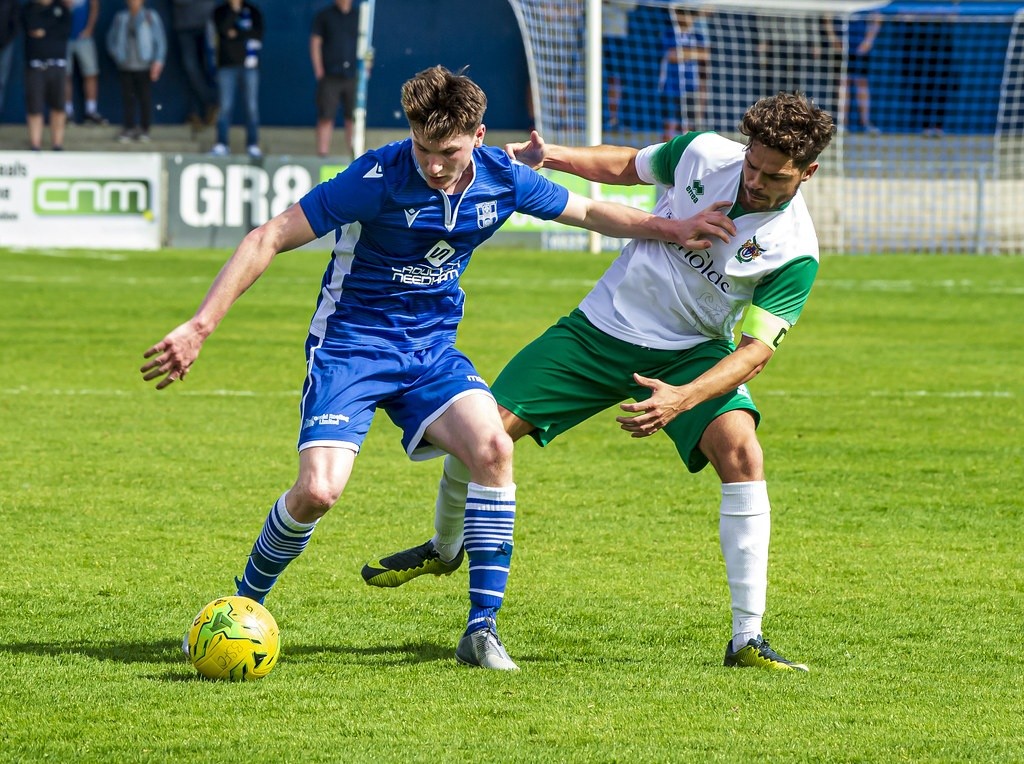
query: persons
66 0 109 127
310 0 372 156
825 12 884 135
554 0 635 128
658 4 717 142
902 16 953 138
361 89 835 672
205 0 266 155
23 0 70 151
106 0 165 142
141 68 736 673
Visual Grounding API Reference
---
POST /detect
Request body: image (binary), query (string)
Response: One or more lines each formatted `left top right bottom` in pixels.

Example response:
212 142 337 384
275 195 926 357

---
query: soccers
186 594 281 682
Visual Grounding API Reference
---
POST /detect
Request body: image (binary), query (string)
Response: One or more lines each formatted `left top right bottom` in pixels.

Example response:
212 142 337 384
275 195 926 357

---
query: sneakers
452 615 522 671
723 634 810 672
360 539 465 589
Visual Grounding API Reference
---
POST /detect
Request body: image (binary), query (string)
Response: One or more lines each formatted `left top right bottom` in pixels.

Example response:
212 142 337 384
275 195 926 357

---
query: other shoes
67 113 110 126
247 144 261 157
213 144 230 158
116 132 152 145
